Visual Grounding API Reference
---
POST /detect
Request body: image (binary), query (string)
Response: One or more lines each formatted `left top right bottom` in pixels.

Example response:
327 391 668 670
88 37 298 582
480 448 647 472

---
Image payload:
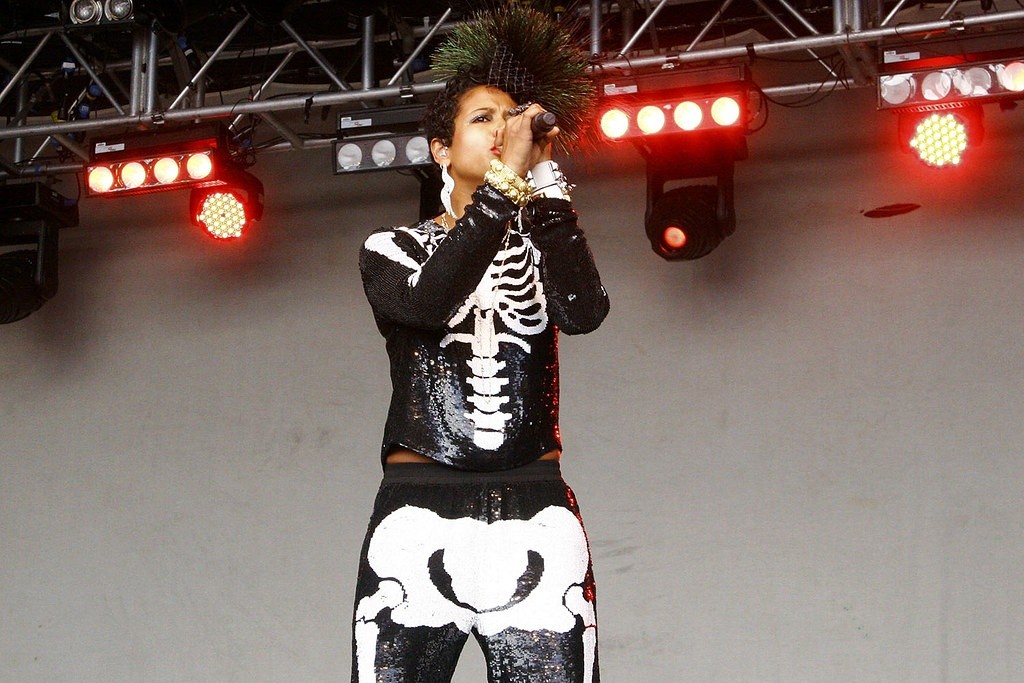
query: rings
508 101 534 115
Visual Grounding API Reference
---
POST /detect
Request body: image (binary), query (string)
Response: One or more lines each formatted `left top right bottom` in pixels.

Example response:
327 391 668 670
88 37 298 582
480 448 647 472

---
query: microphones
530 110 555 143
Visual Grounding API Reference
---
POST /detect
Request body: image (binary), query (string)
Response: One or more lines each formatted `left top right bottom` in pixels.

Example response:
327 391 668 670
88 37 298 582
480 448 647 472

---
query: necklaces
441 211 513 312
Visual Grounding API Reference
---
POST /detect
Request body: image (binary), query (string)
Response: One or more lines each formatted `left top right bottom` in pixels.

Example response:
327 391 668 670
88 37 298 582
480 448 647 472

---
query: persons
361 7 611 683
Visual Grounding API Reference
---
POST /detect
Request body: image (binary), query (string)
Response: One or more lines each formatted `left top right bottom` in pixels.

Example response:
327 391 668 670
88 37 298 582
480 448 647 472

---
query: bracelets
533 184 575 205
491 159 529 193
526 159 567 191
483 171 531 208
